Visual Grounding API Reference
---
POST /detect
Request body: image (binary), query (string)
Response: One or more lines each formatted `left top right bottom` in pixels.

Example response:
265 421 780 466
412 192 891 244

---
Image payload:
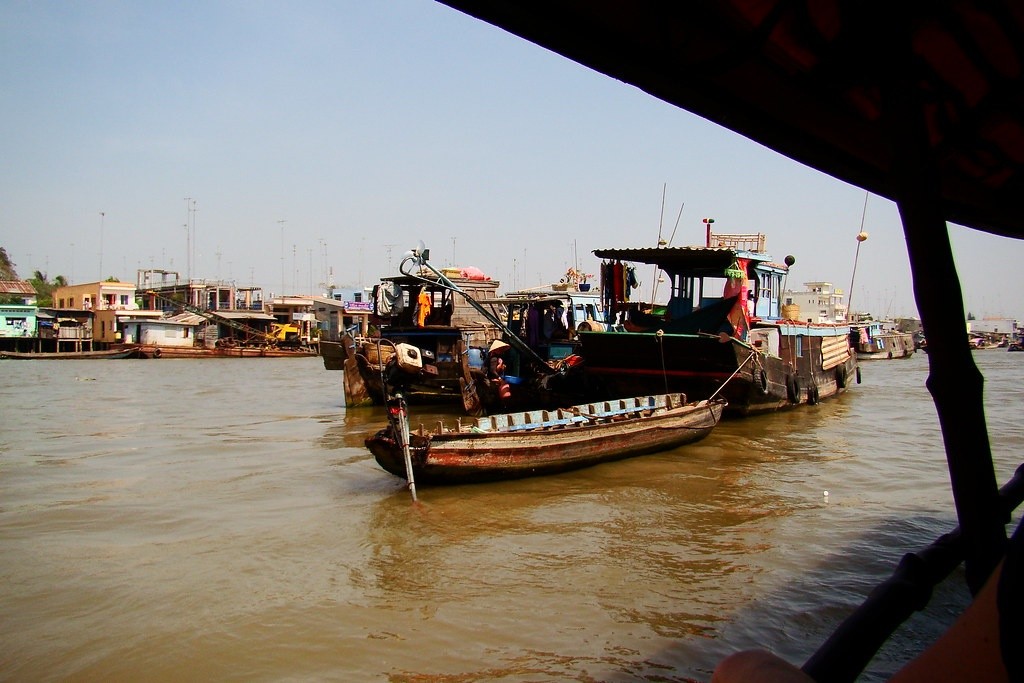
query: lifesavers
787 373 801 404
754 367 770 395
856 367 862 384
888 352 893 359
914 346 917 353
904 350 908 356
808 384 820 406
835 364 847 388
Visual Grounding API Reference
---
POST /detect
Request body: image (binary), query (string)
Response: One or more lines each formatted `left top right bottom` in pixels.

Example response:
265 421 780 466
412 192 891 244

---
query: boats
969 322 1024 354
0 326 140 359
848 310 930 362
365 390 731 481
310 184 870 420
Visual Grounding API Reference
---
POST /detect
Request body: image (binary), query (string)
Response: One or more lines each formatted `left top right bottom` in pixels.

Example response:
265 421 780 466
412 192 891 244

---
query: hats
488 340 511 352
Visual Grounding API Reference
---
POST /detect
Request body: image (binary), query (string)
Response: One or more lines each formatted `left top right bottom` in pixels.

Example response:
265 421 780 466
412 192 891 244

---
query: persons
543 309 555 339
487 339 522 413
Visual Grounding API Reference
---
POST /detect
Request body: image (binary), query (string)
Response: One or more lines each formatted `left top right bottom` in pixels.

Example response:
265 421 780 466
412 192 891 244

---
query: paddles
392 393 425 512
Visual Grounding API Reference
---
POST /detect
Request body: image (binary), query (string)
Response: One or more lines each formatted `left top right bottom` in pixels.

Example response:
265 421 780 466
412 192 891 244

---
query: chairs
418 393 697 436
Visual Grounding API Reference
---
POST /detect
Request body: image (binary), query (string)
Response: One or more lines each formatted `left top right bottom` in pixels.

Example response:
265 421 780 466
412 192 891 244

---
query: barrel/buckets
499 383 511 399
578 321 603 332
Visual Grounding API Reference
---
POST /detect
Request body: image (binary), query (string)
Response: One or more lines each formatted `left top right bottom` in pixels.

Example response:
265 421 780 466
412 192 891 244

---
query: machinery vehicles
146 289 305 350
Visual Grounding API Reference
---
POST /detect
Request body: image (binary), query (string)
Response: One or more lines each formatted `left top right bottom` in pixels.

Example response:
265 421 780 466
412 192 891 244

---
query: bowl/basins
505 376 521 384
